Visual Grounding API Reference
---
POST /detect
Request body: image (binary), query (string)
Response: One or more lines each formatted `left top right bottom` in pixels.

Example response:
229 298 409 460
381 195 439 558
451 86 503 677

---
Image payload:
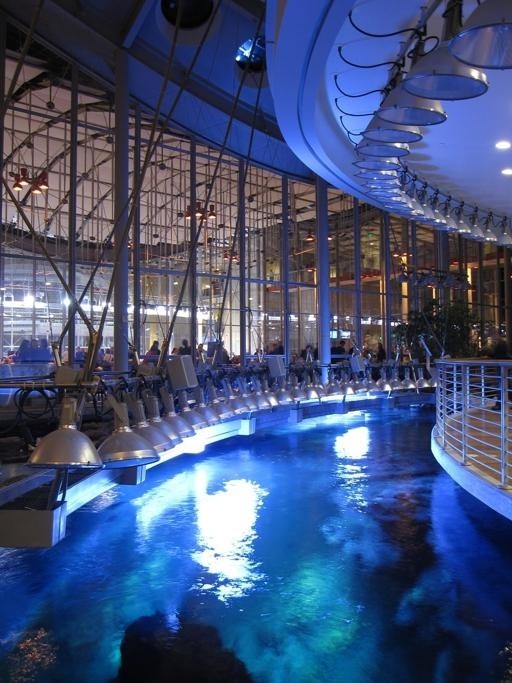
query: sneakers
491 404 501 410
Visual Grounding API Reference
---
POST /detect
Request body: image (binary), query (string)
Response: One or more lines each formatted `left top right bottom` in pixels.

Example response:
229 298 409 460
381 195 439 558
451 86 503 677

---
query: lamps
12 166 50 195
331 0 511 246
21 365 438 473
184 198 217 221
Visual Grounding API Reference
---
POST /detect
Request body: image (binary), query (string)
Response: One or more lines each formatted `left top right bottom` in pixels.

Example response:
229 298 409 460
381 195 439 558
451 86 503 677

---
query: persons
269 341 353 362
377 343 386 362
483 332 511 410
14 338 207 368
